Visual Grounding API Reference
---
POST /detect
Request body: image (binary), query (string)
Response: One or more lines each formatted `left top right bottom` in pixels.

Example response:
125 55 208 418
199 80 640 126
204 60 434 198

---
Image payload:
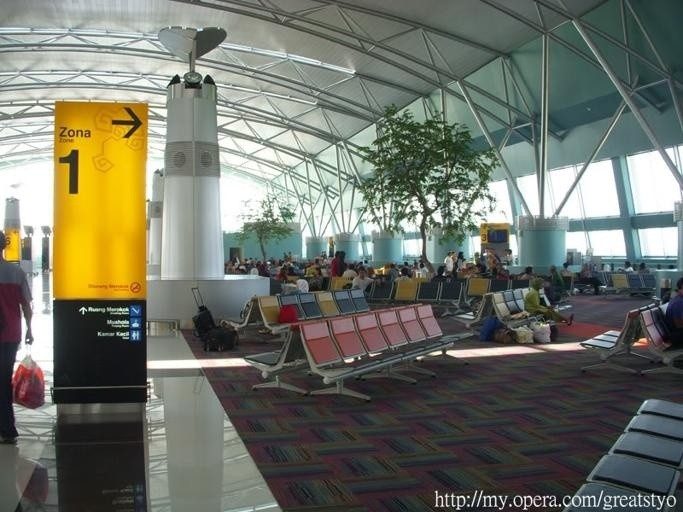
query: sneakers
567 313 575 326
0 436 17 443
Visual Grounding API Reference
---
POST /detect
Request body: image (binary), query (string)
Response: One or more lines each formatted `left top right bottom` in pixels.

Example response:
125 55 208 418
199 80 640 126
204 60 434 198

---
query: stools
562 396 683 512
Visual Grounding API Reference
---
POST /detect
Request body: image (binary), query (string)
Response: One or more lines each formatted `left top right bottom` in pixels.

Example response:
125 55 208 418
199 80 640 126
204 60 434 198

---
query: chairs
218 271 683 403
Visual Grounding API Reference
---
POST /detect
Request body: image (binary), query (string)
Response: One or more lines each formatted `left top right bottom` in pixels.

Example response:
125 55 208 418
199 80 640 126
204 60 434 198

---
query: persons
0 230 35 444
663 276 683 347
521 275 574 326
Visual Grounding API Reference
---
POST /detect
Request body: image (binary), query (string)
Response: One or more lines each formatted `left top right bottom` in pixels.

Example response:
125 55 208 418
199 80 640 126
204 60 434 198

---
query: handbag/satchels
611 274 628 288
479 311 551 344
11 344 44 409
279 304 297 322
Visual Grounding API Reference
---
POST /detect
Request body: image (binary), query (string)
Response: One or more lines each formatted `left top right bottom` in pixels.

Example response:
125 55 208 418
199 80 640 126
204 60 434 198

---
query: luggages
191 286 234 352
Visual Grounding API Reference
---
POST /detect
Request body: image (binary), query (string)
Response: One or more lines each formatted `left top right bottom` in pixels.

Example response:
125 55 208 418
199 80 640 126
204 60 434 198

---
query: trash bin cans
661 278 672 301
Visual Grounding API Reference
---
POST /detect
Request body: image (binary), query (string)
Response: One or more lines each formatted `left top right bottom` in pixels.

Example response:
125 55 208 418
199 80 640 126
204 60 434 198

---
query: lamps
183 69 203 87
